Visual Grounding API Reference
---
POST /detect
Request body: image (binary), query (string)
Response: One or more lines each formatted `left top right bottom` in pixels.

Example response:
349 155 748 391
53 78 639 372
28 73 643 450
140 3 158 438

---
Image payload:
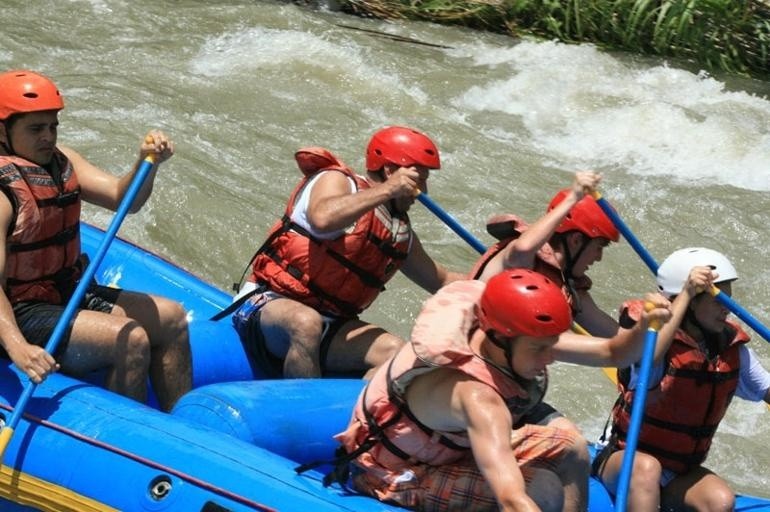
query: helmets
366 126 440 170
655 245 739 299
547 187 620 242
0 70 64 120
478 269 573 337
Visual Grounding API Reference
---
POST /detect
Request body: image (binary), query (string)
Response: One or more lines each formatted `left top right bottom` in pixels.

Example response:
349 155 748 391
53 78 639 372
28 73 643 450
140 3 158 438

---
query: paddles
410 184 618 386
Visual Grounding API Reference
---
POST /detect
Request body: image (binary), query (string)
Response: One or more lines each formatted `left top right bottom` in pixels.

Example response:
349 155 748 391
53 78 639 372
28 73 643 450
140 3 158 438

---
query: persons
462 170 622 431
0 70 192 414
232 126 473 379
595 247 770 512
337 268 674 512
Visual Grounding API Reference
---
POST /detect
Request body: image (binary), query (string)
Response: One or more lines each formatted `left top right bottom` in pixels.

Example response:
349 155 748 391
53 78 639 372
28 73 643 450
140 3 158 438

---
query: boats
0 220 770 512
